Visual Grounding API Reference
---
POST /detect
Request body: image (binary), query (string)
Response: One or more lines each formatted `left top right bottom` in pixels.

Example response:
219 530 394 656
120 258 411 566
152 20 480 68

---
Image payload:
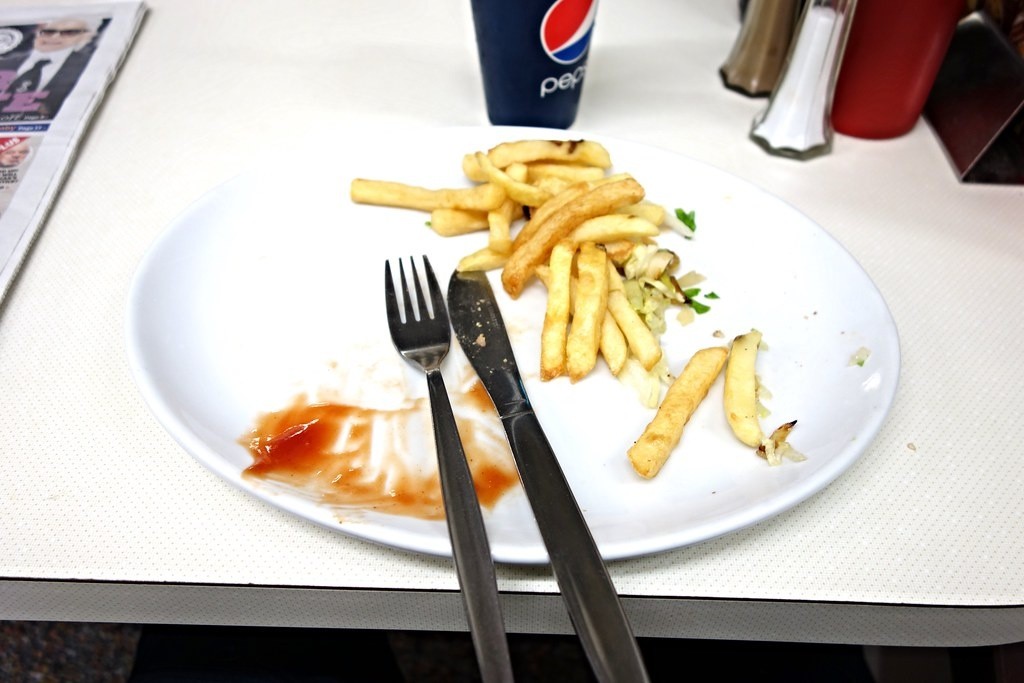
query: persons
0 17 98 122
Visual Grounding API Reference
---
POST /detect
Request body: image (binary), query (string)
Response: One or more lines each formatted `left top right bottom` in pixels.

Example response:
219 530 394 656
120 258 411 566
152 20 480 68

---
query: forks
384 256 514 683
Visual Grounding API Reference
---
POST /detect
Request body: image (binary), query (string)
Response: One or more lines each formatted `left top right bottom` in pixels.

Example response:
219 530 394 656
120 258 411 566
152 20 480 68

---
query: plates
128 131 899 563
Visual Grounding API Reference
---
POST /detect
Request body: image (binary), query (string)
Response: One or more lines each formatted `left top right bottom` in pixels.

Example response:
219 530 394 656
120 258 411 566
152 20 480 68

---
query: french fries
349 139 764 479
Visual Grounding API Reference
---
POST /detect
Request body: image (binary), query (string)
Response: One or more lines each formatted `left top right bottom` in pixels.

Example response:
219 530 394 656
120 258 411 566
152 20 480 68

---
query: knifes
447 270 655 683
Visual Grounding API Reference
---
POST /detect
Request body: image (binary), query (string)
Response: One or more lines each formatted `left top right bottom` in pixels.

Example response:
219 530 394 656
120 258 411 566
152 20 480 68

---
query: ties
5 58 51 95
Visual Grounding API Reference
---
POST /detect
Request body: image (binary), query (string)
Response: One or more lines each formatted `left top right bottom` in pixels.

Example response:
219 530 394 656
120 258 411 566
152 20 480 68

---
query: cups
469 0 598 128
830 0 962 139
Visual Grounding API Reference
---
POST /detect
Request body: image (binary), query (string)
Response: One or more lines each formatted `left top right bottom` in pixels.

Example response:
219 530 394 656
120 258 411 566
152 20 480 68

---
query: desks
0 0 1024 683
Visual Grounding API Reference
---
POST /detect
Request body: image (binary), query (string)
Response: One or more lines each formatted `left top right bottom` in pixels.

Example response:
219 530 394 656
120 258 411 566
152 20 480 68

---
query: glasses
39 29 87 40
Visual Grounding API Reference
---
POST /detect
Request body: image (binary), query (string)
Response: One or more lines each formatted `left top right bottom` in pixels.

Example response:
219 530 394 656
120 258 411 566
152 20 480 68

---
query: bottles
718 0 806 99
752 0 858 159
